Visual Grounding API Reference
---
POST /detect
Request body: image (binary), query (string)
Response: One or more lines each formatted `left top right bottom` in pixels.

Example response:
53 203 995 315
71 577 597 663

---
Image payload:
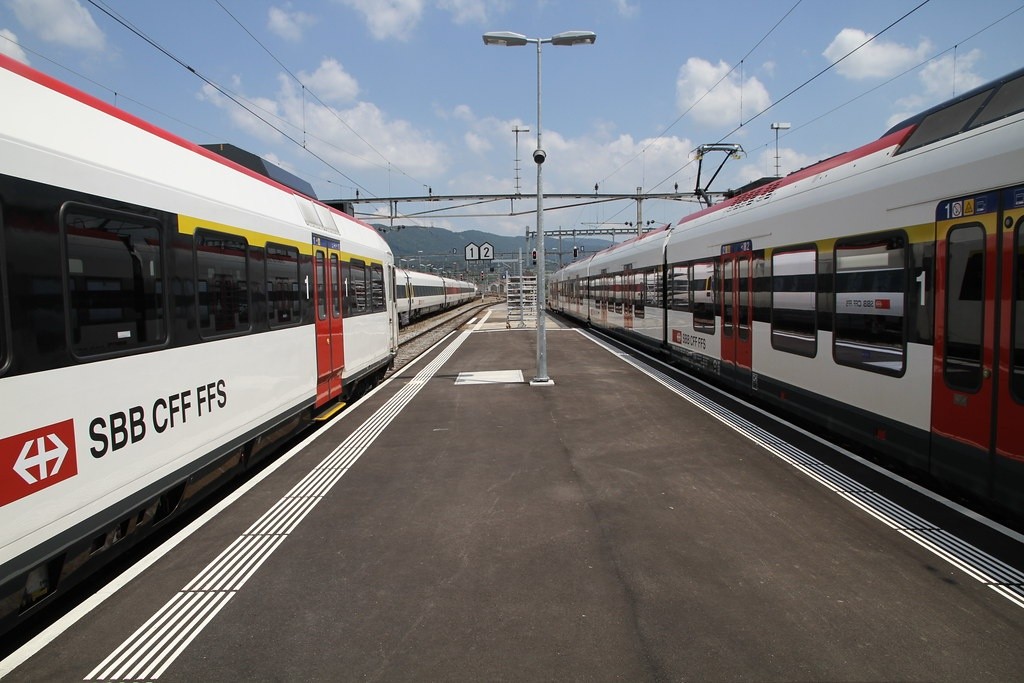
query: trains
395 267 476 328
0 53 397 641
548 67 1024 568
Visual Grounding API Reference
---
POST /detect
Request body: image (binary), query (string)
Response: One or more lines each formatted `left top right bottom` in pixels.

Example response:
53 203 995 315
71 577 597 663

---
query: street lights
482 28 597 387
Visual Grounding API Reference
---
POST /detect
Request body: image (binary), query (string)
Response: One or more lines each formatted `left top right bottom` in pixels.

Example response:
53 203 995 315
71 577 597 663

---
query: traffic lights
573 248 579 258
532 251 537 260
